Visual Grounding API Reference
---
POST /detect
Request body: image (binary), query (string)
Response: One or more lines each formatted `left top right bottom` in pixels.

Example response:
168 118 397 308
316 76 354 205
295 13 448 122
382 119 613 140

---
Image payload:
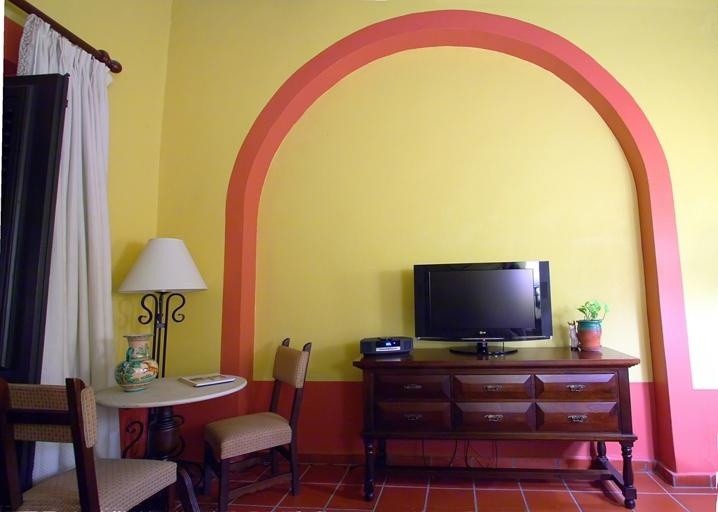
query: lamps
117 238 203 498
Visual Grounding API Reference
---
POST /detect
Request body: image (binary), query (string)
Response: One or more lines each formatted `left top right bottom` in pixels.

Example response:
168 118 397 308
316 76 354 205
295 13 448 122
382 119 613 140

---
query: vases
114 335 158 392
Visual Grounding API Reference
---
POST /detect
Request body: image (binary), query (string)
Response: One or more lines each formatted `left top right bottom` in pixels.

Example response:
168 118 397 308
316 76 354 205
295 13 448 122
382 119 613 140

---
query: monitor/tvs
414 261 553 355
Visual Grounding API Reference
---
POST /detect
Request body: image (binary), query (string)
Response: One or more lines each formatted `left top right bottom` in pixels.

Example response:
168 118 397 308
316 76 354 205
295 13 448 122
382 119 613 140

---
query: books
181 372 235 387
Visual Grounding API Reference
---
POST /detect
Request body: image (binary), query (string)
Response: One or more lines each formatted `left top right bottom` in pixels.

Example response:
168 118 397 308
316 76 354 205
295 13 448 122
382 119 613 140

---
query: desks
352 347 641 510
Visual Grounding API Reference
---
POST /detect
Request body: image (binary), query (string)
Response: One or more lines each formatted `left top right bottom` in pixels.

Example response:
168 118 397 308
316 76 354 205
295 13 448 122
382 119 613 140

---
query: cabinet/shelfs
373 374 620 433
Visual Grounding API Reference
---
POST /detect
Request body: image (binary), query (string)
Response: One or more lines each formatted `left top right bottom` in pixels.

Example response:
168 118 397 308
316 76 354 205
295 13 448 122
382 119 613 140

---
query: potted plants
576 301 610 350
